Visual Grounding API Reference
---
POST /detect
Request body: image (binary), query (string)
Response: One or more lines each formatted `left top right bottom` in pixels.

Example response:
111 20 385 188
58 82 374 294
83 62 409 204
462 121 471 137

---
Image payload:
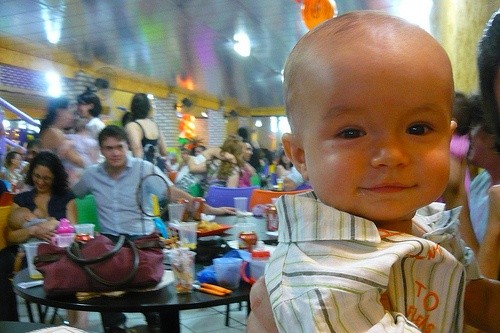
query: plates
267 230 279 237
197 225 235 237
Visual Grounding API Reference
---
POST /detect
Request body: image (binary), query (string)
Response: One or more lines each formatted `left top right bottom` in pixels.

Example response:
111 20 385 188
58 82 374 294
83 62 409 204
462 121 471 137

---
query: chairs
246 189 310 212
206 185 259 208
75 195 100 232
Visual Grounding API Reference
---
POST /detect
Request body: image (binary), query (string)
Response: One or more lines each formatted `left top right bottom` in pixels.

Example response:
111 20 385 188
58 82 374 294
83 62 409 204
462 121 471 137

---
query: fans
85 67 117 100
174 94 198 111
224 107 241 122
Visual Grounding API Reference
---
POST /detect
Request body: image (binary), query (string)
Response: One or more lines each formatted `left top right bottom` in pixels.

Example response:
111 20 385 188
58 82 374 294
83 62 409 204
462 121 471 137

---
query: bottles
249 241 270 281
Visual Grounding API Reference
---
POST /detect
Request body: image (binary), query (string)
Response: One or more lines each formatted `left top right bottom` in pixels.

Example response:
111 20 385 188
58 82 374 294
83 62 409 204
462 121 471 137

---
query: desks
12 256 252 333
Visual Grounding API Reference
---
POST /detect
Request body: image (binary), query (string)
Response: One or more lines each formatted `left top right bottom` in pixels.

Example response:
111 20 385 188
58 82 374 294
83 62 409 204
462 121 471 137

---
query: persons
177 136 305 198
265 11 500 333
0 150 27 196
72 90 106 140
7 207 62 274
71 126 239 332
31 95 85 187
122 91 168 171
3 146 88 333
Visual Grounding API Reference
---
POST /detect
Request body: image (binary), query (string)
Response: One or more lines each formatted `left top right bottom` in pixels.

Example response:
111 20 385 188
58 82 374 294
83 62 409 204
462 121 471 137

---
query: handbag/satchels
34 232 164 296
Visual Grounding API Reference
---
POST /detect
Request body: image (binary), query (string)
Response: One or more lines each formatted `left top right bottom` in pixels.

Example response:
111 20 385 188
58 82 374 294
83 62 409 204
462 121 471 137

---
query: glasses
30 173 53 183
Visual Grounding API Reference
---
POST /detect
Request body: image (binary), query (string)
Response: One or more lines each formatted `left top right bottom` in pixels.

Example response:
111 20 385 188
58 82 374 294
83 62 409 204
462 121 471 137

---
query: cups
57 233 75 247
168 251 197 294
75 224 95 236
23 243 49 278
213 258 243 290
168 204 184 224
277 178 284 191
178 222 197 250
233 197 248 218
